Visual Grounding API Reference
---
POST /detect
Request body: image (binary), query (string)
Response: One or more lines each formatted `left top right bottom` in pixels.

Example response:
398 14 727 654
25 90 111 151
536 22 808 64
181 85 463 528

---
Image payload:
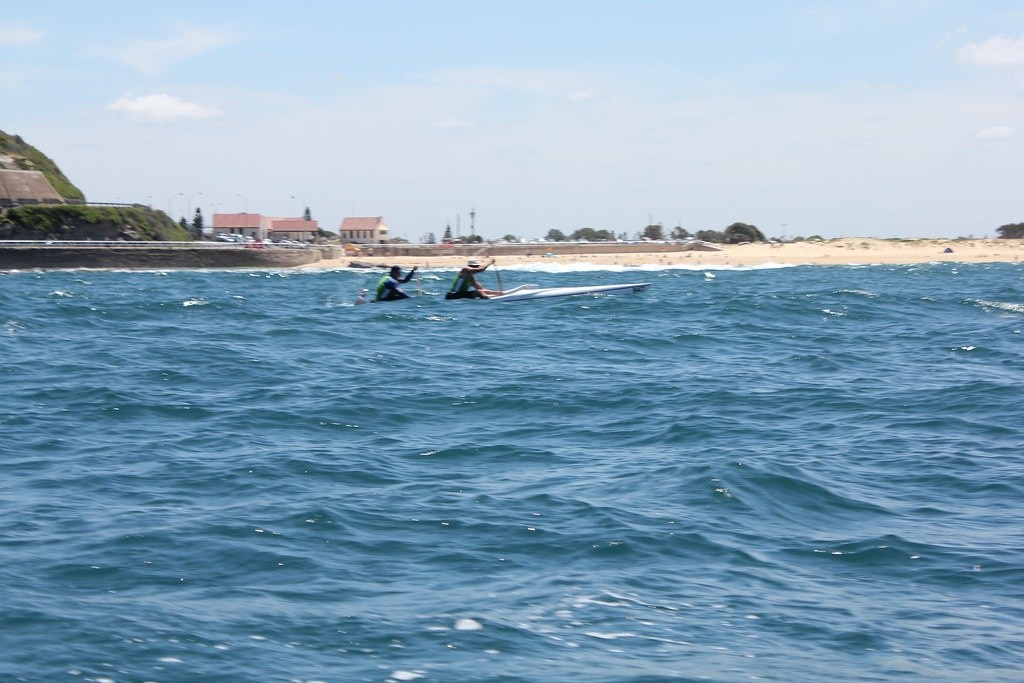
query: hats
467 260 481 266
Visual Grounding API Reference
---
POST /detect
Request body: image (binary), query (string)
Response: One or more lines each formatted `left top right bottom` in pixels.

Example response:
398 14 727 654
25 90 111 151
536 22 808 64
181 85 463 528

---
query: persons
375 266 417 301
447 258 502 299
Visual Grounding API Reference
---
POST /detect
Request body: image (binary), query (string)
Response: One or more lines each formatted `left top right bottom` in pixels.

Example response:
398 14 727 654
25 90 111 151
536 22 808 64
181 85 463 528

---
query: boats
483 282 652 300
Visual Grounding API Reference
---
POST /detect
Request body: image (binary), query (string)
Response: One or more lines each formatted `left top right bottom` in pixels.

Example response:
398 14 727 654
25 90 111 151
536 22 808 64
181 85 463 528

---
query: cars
213 232 328 246
